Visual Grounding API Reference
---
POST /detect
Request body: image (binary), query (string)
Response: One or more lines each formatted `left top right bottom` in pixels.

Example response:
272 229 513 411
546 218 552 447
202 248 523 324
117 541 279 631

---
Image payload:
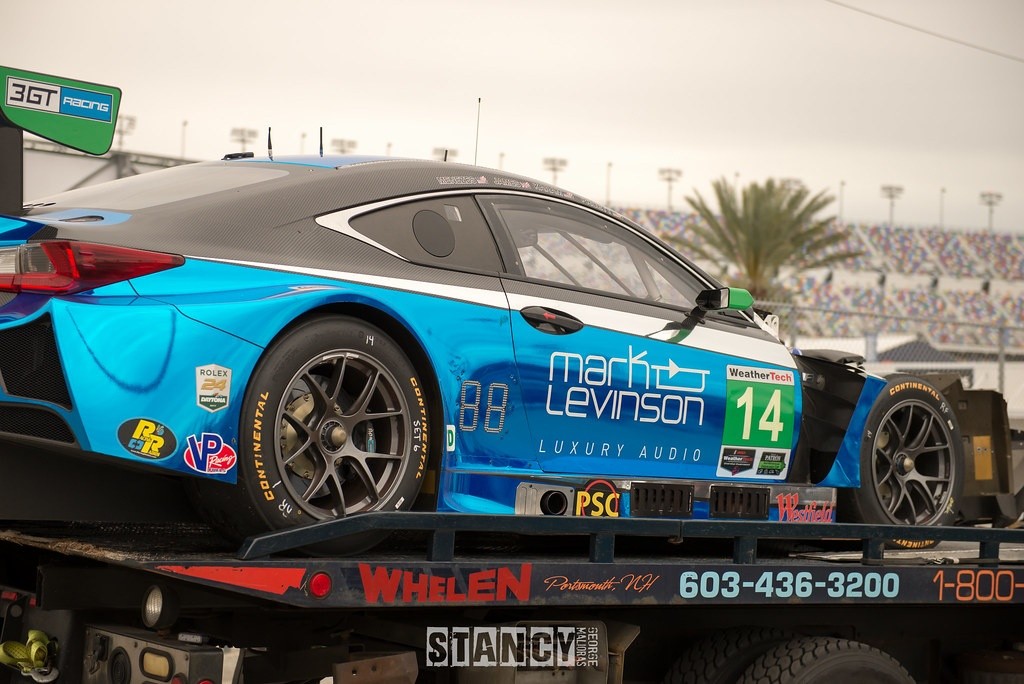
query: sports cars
0 159 964 548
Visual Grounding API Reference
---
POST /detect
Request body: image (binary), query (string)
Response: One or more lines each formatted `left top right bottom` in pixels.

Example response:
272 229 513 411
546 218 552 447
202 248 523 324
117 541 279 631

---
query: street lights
839 179 847 227
883 185 904 223
115 115 257 160
940 187 947 231
605 163 612 205
979 191 1003 229
543 156 567 185
331 138 508 169
658 168 684 209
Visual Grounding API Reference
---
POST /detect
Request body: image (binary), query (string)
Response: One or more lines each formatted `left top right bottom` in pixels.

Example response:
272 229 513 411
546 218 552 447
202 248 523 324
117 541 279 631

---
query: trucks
2 522 1024 683
